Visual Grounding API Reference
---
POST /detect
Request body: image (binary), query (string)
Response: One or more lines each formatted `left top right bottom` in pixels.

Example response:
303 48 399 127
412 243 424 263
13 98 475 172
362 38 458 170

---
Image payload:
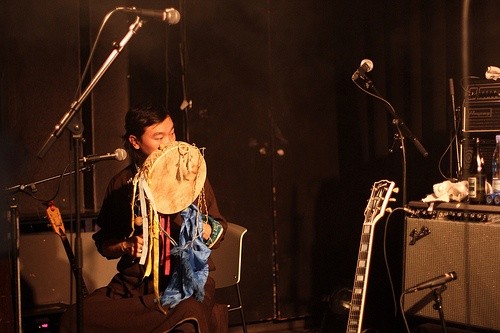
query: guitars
346 179 400 333
45 203 89 297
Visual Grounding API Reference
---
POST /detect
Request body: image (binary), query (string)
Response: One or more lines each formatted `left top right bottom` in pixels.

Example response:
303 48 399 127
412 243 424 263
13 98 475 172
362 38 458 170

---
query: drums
143 140 207 214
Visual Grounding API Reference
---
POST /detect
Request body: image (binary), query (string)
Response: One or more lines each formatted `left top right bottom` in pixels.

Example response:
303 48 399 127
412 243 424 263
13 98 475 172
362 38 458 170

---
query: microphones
115 7 182 26
79 148 127 163
352 59 374 81
402 208 420 218
403 271 458 294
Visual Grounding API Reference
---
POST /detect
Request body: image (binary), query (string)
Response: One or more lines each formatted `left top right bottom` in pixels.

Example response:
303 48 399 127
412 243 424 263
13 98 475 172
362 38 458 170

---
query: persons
79 97 227 333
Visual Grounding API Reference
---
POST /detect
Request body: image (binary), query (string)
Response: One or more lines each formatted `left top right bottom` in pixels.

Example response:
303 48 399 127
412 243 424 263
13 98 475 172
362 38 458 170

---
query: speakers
402 203 500 333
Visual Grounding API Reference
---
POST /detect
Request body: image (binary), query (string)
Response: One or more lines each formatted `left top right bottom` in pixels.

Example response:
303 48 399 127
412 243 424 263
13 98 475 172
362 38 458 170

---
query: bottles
468 137 487 205
492 135 500 193
